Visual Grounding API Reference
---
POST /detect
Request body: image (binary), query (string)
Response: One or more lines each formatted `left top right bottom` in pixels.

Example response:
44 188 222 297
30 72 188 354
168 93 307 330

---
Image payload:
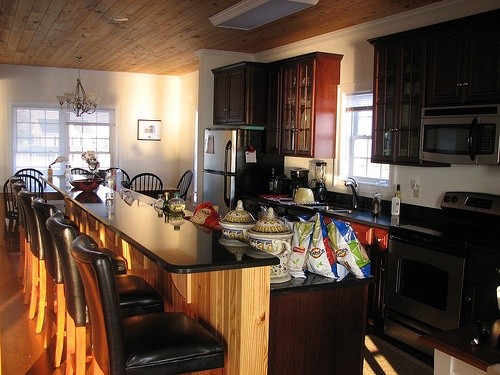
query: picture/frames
136 119 162 140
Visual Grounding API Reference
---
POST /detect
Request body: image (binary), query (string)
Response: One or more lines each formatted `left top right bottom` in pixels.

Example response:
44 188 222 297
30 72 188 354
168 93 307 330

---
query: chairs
2 168 224 375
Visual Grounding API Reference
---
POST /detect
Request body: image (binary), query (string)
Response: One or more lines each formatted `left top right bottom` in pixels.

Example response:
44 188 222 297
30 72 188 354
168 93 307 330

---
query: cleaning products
395 184 401 202
371 192 382 214
390 193 401 216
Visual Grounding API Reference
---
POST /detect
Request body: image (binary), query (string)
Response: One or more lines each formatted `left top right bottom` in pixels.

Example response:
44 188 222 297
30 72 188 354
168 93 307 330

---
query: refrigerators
201 128 284 210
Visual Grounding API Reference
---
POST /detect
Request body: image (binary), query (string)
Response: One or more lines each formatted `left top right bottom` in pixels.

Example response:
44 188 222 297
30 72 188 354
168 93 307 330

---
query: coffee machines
290 167 309 198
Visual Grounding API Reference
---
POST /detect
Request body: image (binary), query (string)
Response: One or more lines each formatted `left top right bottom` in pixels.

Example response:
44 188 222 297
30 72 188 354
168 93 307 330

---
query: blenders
312 161 328 203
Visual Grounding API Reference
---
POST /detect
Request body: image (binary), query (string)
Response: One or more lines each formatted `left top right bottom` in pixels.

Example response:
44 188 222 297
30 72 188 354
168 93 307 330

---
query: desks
38 172 281 375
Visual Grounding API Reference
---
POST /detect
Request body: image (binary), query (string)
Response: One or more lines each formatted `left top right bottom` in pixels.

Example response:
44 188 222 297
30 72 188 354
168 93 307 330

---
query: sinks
330 210 372 213
305 205 346 211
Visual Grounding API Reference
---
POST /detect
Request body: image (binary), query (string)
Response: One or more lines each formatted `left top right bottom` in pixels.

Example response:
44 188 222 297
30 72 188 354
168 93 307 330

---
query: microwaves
419 104 500 166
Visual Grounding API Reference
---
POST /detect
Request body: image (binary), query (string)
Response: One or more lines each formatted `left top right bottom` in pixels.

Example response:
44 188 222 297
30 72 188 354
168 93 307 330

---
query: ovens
382 234 475 331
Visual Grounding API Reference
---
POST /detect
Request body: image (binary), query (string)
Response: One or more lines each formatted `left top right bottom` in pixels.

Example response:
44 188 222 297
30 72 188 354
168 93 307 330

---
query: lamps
208 0 319 30
56 55 102 118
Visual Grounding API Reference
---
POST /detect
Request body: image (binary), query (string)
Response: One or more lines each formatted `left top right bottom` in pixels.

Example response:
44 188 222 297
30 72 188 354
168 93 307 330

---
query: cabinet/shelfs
209 51 345 160
323 216 389 334
365 7 500 169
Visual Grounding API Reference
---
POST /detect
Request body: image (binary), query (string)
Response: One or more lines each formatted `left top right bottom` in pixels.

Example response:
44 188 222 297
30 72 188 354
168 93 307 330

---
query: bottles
371 197 379 217
65 164 72 179
269 167 275 195
218 200 256 243
156 192 186 213
396 183 401 198
373 192 381 211
391 193 400 215
48 165 52 176
245 208 295 283
108 170 115 188
116 169 123 192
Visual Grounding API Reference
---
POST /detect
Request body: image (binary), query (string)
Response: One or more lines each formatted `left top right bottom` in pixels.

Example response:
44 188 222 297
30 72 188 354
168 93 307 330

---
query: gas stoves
387 191 500 257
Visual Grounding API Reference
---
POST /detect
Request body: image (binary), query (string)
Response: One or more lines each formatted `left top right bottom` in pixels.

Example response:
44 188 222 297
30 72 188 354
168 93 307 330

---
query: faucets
344 176 360 209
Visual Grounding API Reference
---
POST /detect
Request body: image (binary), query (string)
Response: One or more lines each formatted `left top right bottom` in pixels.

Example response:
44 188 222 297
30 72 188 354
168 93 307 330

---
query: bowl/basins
294 188 314 203
